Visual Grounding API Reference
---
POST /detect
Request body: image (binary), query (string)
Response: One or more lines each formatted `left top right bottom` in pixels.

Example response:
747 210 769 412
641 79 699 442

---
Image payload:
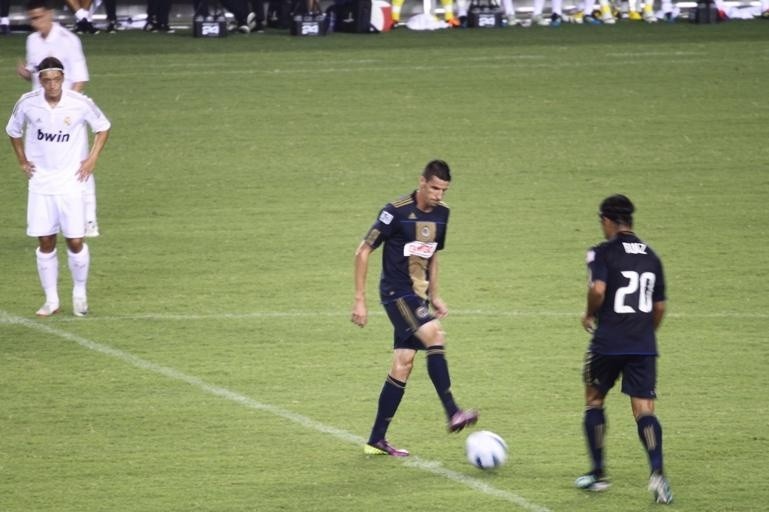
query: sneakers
646 475 675 506
574 472 613 493
363 438 411 458
36 299 60 317
445 407 480 435
74 18 101 36
70 294 88 318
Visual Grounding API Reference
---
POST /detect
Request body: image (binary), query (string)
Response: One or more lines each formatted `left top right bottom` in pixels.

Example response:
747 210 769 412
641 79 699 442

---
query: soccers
466 431 507 468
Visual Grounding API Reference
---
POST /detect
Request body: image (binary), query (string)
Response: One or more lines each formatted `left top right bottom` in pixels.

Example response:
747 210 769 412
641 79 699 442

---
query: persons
17 3 90 93
6 55 111 316
350 160 478 456
574 194 674 506
